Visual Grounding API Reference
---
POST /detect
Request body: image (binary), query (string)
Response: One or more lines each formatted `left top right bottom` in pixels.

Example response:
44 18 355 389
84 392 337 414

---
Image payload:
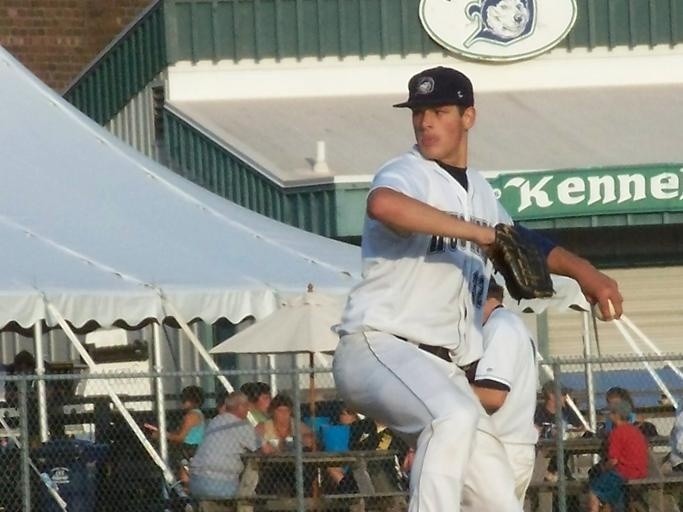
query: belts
389 334 454 367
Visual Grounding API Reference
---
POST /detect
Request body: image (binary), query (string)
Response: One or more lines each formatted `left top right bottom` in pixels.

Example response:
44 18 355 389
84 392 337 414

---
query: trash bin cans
36 440 112 512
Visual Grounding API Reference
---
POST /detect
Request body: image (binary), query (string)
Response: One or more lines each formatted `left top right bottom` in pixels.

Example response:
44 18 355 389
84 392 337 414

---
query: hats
541 380 569 394
391 66 475 110
598 400 633 418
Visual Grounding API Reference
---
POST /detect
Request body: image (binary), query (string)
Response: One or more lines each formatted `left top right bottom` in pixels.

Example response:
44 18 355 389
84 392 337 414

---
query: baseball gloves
485 223 553 303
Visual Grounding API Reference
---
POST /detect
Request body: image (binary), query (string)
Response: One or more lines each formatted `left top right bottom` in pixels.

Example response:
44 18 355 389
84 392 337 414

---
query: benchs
523 435 683 511
175 435 411 512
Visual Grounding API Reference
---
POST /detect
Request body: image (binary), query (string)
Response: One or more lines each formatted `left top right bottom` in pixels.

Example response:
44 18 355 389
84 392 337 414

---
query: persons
332 66 624 511
470 275 541 511
